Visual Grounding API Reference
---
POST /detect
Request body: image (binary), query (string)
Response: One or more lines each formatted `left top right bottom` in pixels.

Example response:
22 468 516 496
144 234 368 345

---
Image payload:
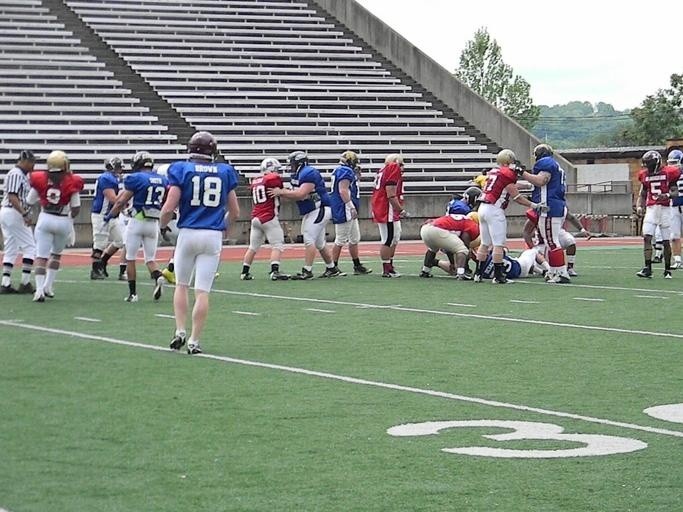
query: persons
635 149 681 279
665 149 683 270
88 151 224 284
1 148 38 296
25 149 81 303
639 159 664 264
419 143 582 286
237 151 410 282
159 132 241 355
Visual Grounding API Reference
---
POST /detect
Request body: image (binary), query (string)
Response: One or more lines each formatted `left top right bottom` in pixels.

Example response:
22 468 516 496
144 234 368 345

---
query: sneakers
652 254 663 263
545 272 555 280
568 269 578 276
118 271 128 281
353 265 372 274
419 271 433 277
241 273 255 281
293 268 314 280
123 294 138 301
170 334 186 349
492 277 515 284
18 282 35 293
547 274 570 284
474 274 482 283
382 271 402 278
187 345 202 354
335 266 347 276
671 261 682 269
318 267 340 278
153 276 164 301
0 284 18 294
92 259 109 280
32 288 56 301
664 270 672 279
270 270 292 281
162 268 175 285
636 267 653 278
455 274 473 280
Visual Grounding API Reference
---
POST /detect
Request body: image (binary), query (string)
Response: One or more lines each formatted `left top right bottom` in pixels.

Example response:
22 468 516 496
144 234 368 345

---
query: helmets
496 149 517 166
287 151 309 175
189 131 219 160
384 153 404 168
104 155 125 173
533 143 554 161
466 211 480 224
47 149 69 172
260 157 283 173
667 150 683 163
464 187 483 207
131 151 154 171
640 150 662 176
339 151 360 171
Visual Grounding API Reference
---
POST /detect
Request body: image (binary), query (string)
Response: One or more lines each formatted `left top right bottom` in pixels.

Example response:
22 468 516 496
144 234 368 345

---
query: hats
18 149 40 161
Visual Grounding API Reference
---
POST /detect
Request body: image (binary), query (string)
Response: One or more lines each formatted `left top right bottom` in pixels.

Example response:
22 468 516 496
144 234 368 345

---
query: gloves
159 227 172 244
399 209 410 220
509 163 524 177
103 213 111 224
531 202 549 212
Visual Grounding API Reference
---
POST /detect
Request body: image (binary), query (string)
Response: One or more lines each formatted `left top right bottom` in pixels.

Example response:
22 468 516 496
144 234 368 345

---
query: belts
5 205 13 208
